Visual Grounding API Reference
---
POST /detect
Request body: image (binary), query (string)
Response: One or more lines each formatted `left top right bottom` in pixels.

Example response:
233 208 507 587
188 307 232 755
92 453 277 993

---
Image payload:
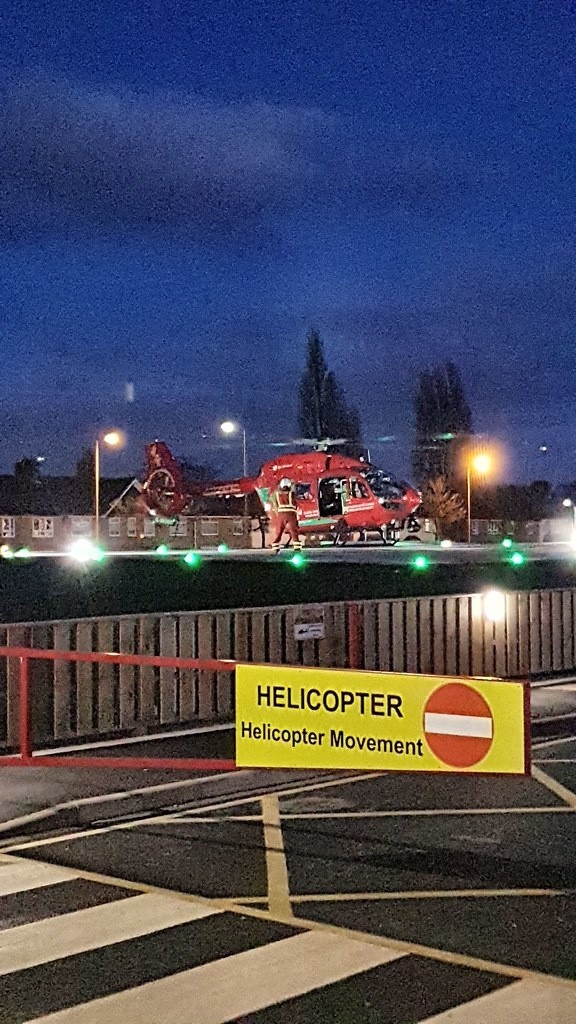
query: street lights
93 430 119 547
221 419 249 549
466 449 492 544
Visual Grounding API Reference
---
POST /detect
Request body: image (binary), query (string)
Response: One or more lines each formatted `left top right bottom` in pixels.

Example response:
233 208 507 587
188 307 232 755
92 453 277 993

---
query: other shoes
294 542 303 550
272 543 280 550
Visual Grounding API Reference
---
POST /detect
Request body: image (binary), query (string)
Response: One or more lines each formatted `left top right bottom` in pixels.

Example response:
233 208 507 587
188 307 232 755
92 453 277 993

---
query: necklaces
270 476 307 556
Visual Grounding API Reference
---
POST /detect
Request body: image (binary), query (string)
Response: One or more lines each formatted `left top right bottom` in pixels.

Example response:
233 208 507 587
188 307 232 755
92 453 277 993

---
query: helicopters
144 428 424 553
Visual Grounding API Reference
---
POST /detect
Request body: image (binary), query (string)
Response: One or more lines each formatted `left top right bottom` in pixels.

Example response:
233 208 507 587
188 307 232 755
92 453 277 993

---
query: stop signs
233 662 527 775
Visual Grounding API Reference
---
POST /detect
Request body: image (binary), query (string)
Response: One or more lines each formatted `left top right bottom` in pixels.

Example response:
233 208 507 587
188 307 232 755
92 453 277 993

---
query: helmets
280 477 291 489
